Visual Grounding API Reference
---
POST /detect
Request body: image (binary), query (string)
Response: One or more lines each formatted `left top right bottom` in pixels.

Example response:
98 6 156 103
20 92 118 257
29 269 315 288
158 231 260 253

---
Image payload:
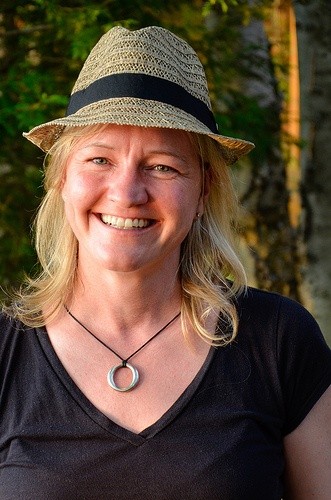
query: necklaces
62 297 179 391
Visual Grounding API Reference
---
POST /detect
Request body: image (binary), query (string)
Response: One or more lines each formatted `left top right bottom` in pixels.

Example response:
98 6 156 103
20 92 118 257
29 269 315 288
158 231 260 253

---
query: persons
0 24 331 500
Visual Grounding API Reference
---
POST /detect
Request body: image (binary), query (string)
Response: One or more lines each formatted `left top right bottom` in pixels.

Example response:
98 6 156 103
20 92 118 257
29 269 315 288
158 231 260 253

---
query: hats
22 25 255 166
